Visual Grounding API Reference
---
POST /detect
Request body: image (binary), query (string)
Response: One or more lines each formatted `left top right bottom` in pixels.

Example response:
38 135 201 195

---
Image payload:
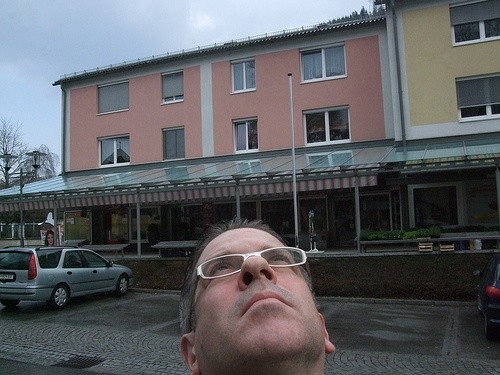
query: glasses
192 247 311 289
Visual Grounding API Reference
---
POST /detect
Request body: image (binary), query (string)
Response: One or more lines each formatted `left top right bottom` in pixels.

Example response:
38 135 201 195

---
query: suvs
1 245 134 310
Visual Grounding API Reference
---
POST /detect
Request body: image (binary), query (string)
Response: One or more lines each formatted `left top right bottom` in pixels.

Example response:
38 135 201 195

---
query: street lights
0 150 47 247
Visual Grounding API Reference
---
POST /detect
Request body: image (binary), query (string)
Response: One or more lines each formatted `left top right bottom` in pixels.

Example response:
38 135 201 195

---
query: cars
472 250 500 338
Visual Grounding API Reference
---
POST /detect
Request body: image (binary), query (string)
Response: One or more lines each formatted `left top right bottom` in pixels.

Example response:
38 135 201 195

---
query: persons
45 230 55 247
180 218 336 375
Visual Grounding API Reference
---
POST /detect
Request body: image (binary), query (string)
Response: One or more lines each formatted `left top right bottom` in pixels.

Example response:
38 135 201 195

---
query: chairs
40 253 57 267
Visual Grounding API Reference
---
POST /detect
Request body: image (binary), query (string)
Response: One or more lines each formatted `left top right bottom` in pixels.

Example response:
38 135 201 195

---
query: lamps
81 208 88 218
120 209 126 218
